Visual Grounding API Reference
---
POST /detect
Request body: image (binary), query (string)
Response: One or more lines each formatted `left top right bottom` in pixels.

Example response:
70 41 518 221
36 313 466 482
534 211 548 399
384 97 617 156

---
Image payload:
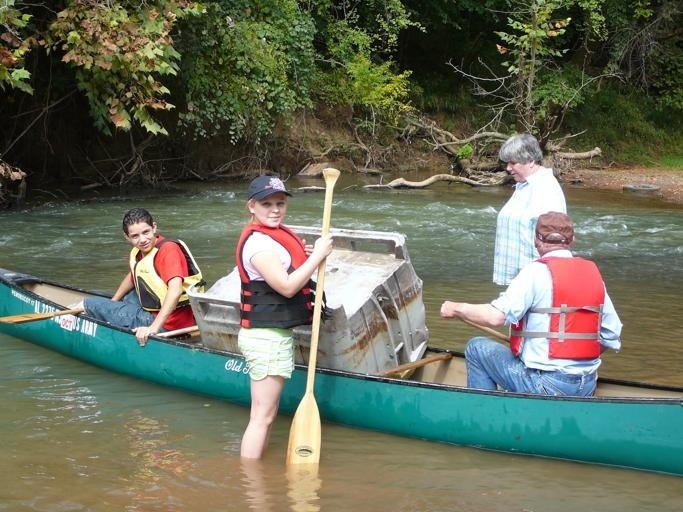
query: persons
440 212 624 396
493 133 570 288
237 173 336 461
77 206 207 349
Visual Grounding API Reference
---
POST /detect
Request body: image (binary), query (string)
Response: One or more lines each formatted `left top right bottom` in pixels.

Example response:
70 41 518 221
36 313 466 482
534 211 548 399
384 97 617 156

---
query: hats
246 174 294 202
535 211 575 245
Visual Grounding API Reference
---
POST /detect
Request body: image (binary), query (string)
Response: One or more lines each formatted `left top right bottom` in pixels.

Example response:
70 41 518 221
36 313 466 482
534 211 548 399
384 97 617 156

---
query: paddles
0 309 83 323
284 167 340 465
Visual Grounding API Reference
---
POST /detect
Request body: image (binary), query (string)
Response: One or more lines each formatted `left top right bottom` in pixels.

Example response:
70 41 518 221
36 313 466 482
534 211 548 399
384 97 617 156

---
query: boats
0 265 681 475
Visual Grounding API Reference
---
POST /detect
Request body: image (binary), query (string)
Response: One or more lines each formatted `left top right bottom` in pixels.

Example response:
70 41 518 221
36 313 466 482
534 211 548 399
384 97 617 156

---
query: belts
533 368 597 384
